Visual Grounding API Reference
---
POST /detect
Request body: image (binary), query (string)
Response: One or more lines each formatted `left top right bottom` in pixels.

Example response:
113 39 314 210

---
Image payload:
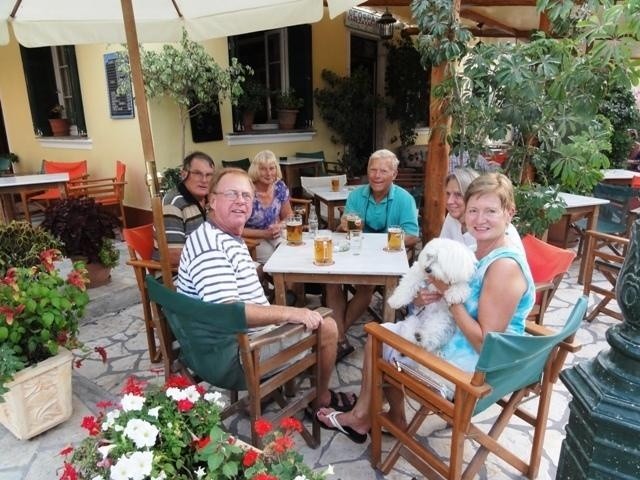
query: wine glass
346 216 357 240
294 204 306 236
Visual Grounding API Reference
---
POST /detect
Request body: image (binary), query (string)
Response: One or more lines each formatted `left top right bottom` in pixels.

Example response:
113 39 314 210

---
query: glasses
214 190 252 201
466 207 502 217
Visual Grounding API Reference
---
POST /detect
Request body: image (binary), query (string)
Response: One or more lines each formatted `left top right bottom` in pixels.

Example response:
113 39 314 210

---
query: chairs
248 197 314 308
363 296 589 480
222 158 251 178
67 162 127 232
297 151 328 184
522 232 579 326
122 223 261 365
298 176 348 216
323 160 361 185
143 273 334 450
28 159 88 214
322 205 415 325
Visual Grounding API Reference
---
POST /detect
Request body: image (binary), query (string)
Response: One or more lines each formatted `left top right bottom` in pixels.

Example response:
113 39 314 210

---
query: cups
314 229 334 262
330 176 340 193
387 225 405 250
350 229 362 255
279 214 304 244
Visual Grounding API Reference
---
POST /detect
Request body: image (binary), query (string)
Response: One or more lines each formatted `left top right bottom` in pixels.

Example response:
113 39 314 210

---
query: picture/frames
102 49 135 120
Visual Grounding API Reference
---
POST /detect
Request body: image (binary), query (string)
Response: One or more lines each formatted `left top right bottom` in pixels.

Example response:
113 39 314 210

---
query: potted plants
47 104 71 136
274 89 306 130
234 84 269 132
36 196 120 289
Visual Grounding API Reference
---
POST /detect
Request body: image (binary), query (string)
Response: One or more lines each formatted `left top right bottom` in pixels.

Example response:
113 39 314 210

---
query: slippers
313 407 368 444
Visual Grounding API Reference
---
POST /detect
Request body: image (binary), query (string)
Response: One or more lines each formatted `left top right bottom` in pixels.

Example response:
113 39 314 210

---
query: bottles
308 205 319 238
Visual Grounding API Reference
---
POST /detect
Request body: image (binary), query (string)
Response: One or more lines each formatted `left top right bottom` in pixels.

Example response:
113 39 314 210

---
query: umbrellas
0 1 369 288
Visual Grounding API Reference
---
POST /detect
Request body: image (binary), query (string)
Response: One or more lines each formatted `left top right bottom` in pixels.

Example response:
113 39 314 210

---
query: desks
0 172 71 230
574 168 638 227
525 190 611 284
268 156 322 197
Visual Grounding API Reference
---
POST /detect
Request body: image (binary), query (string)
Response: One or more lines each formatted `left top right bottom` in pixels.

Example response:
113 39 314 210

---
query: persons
175 166 358 420
322 148 420 364
244 149 297 307
408 167 527 319
315 171 537 444
151 150 216 291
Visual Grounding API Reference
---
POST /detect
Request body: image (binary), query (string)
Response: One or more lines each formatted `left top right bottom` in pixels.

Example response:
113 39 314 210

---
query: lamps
375 7 396 45
580 228 633 323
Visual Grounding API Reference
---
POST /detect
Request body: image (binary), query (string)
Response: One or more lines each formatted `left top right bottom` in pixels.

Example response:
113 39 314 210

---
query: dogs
387 236 478 361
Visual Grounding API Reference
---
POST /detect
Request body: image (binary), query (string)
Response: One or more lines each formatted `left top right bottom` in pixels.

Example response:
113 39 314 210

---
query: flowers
0 248 107 395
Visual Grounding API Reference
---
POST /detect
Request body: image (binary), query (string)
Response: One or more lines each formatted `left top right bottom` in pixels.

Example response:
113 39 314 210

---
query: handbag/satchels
394 360 448 400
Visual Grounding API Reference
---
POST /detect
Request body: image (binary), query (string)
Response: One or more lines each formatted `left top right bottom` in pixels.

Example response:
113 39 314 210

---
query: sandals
334 335 355 365
305 388 358 418
241 385 283 417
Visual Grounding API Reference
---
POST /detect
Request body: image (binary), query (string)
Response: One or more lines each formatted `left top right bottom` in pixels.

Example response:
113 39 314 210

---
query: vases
0 344 77 442
